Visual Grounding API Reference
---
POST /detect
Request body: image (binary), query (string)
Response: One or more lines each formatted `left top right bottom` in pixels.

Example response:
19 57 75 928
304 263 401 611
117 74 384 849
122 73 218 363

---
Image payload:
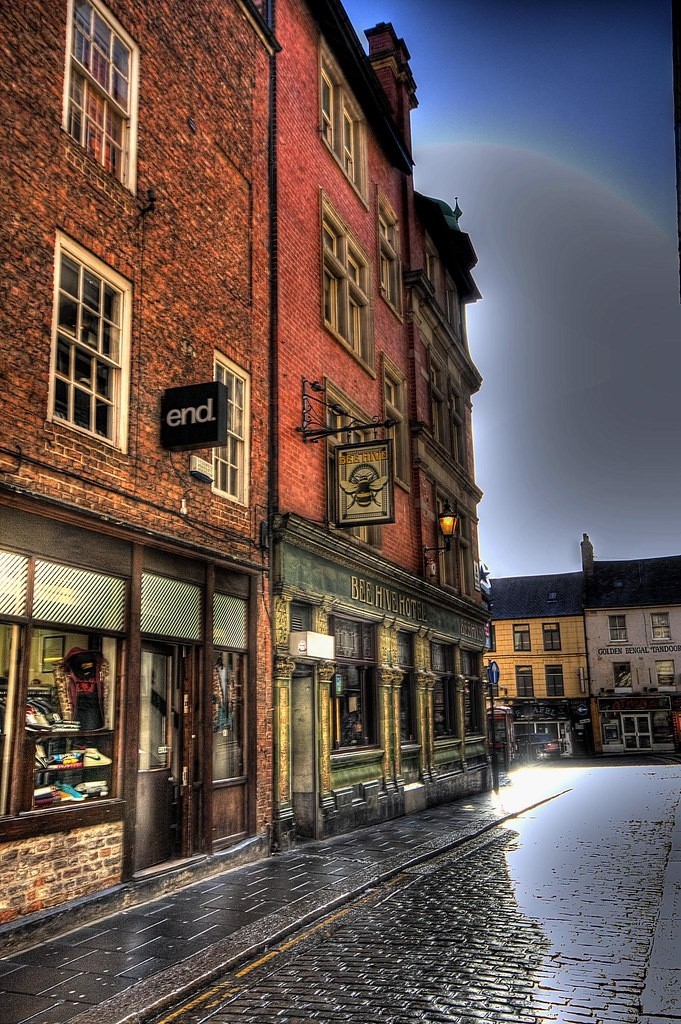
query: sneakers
54 781 87 802
36 713 53 733
82 751 112 766
24 711 41 733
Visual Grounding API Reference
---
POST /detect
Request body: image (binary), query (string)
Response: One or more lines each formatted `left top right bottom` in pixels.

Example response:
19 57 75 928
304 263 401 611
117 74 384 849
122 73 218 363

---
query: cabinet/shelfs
23 729 114 810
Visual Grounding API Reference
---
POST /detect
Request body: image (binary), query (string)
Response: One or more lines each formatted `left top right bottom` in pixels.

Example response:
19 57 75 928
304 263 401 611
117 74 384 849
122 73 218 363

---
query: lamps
423 497 460 568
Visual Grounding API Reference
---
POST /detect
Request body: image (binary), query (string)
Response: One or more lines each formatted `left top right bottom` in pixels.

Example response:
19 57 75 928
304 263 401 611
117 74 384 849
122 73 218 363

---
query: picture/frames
41 635 66 673
